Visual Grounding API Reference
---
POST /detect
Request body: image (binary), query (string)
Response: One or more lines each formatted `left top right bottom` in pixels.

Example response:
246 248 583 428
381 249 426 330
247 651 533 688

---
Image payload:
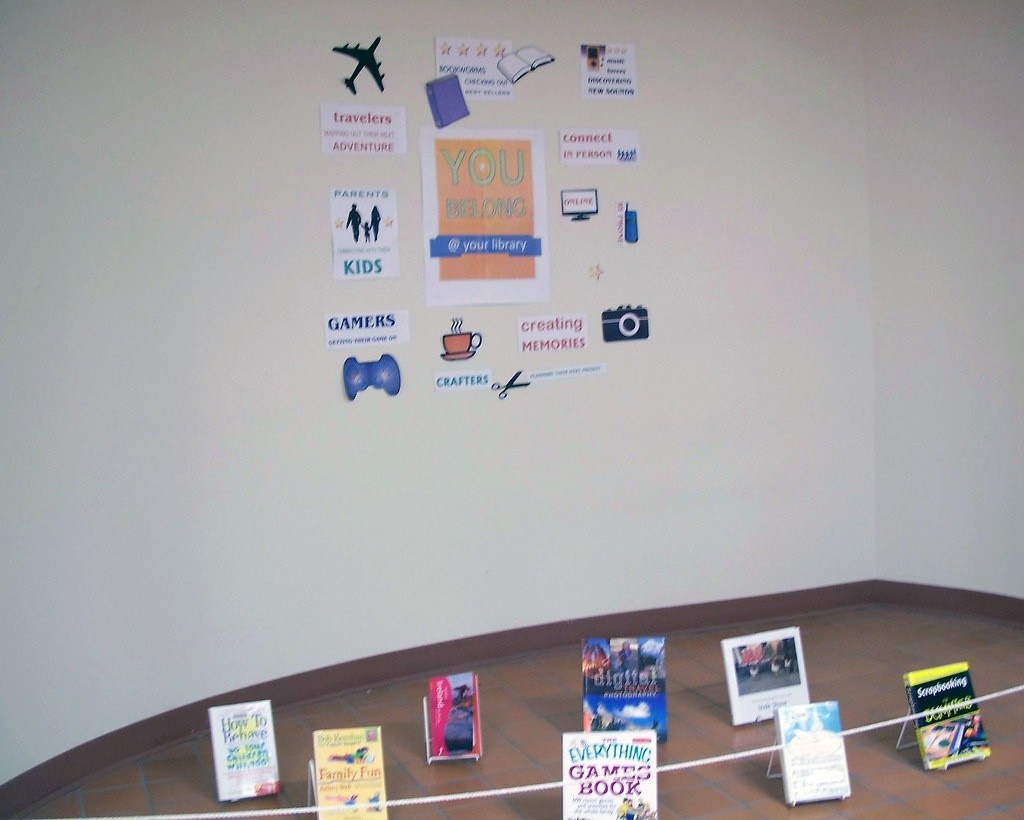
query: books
559 731 659 820
581 635 667 744
312 725 389 820
207 699 280 801
773 699 853 808
903 661 992 771
429 671 484 759
721 626 810 727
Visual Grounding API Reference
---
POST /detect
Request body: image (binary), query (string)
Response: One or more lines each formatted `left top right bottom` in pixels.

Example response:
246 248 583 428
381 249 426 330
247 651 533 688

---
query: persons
617 640 632 672
